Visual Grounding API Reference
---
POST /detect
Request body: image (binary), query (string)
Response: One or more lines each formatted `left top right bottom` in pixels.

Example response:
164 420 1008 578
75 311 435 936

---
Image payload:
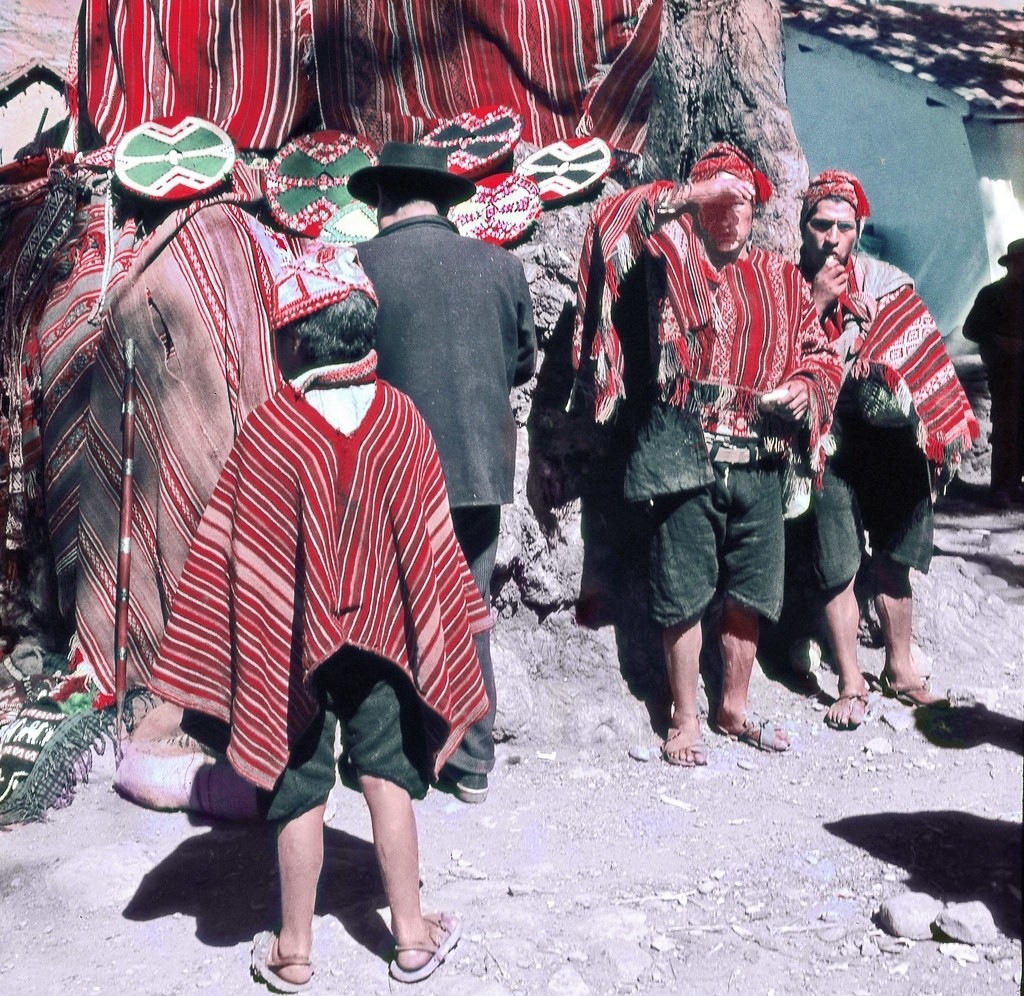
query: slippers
714 711 792 753
879 667 945 708
826 676 869 730
251 916 316 993
663 715 709 768
390 911 464 983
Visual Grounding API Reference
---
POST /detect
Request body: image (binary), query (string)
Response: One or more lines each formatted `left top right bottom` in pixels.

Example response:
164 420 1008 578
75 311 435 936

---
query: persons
794 168 982 730
564 143 844 767
338 142 540 805
961 238 1024 503
148 245 497 993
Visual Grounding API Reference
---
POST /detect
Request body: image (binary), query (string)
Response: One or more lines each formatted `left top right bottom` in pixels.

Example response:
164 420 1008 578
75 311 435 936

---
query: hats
800 169 872 240
687 142 771 205
347 140 479 209
271 244 379 331
998 238 1024 265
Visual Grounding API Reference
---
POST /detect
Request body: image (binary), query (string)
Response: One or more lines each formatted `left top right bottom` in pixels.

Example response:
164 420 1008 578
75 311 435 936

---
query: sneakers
439 763 491 804
